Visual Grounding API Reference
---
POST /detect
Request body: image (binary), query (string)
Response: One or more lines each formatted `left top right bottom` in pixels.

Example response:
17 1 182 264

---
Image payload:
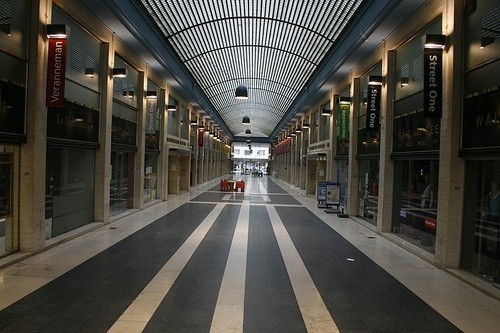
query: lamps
235 85 249 100
320 109 331 116
245 128 251 135
122 91 134 99
338 96 352 105
245 136 253 154
46 24 71 40
110 68 128 80
199 125 231 148
146 90 158 100
167 104 177 111
190 120 198 125
271 123 310 149
401 77 410 86
420 33 447 50
85 68 95 77
366 75 383 86
242 115 250 124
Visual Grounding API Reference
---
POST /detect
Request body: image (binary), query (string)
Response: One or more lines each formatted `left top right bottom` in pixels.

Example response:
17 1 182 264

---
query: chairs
219 179 244 192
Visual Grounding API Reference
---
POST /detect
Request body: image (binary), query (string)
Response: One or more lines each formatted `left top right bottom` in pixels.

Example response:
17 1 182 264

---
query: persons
420 174 435 246
479 180 500 282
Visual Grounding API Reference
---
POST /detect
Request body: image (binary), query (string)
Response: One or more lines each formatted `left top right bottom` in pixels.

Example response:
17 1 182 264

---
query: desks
223 180 235 192
